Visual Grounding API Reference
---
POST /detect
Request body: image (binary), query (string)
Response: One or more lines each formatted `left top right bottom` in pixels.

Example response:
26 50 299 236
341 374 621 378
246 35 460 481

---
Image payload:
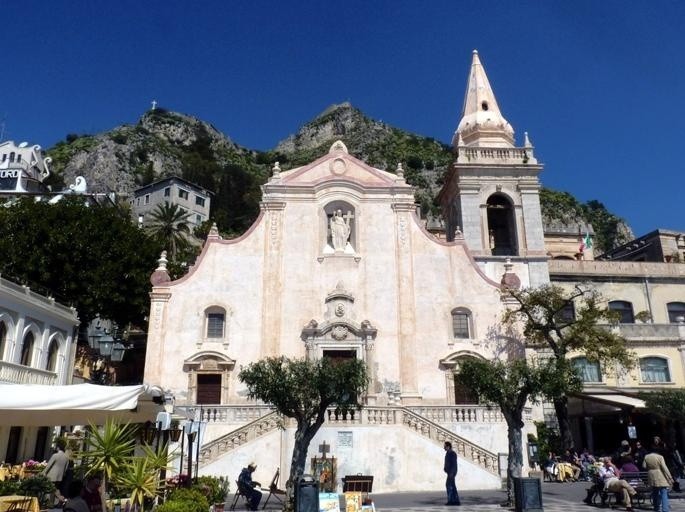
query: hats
249 462 257 469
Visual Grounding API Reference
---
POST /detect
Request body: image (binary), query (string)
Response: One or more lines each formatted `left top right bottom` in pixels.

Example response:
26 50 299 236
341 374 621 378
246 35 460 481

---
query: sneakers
631 494 643 499
244 503 259 511
626 507 635 512
444 501 461 505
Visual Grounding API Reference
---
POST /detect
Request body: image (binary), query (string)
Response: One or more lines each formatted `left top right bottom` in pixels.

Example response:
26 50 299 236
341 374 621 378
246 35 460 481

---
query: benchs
594 472 655 506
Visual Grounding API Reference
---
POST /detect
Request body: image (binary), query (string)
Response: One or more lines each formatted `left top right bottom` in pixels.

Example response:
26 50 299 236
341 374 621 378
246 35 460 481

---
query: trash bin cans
292 474 320 512
513 477 545 512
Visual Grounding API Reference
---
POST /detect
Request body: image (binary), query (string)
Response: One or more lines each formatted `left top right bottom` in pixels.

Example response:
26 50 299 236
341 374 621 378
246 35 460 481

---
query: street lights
89 325 127 385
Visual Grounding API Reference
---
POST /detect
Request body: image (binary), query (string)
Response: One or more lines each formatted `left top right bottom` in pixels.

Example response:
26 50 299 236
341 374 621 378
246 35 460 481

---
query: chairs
230 478 251 511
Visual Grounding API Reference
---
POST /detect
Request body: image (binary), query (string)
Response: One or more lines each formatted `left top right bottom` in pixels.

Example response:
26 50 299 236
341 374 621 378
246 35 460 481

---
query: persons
598 437 674 512
443 442 461 506
546 450 596 482
40 440 70 489
330 209 349 252
239 463 262 511
